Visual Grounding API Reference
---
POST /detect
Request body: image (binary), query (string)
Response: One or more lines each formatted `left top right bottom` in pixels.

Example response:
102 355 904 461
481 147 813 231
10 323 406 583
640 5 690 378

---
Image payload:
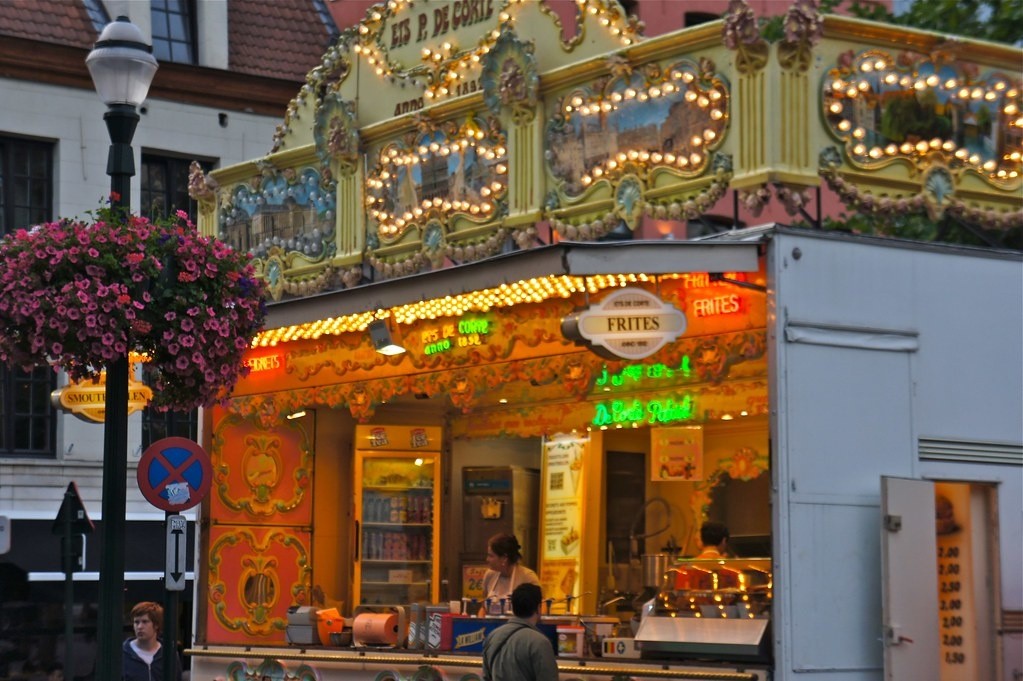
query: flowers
0 191 269 416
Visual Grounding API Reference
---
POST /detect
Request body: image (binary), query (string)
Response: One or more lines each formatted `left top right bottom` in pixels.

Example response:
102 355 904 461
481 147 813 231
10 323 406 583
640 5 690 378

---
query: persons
689 520 738 570
480 581 560 681
120 601 182 681
476 532 541 621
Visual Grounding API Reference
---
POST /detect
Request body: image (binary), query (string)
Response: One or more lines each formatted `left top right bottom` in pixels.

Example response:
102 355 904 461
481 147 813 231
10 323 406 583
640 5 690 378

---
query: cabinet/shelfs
349 425 442 617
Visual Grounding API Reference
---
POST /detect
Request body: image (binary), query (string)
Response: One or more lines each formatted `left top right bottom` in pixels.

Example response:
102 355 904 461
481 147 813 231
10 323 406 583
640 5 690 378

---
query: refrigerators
345 423 442 613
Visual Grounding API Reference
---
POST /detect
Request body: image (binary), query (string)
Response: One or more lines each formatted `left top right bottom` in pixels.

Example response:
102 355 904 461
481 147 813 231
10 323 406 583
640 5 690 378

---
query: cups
317 617 344 647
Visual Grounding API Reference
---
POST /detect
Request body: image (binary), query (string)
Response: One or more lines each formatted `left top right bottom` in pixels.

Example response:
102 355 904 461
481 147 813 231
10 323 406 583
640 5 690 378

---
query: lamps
367 307 407 356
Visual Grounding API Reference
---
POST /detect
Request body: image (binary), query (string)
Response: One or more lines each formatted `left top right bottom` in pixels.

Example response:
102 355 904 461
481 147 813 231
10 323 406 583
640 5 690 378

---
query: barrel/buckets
556 625 586 658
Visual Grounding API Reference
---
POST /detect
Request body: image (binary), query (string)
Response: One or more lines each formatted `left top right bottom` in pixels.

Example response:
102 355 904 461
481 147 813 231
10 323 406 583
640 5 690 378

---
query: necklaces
701 549 720 556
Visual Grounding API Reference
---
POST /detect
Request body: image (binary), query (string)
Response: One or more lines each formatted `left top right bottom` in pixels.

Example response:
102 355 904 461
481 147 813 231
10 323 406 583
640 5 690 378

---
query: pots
640 554 671 588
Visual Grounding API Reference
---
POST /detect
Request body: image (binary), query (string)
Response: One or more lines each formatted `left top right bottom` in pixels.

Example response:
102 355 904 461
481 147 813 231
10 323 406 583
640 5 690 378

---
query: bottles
362 530 428 560
361 495 431 523
